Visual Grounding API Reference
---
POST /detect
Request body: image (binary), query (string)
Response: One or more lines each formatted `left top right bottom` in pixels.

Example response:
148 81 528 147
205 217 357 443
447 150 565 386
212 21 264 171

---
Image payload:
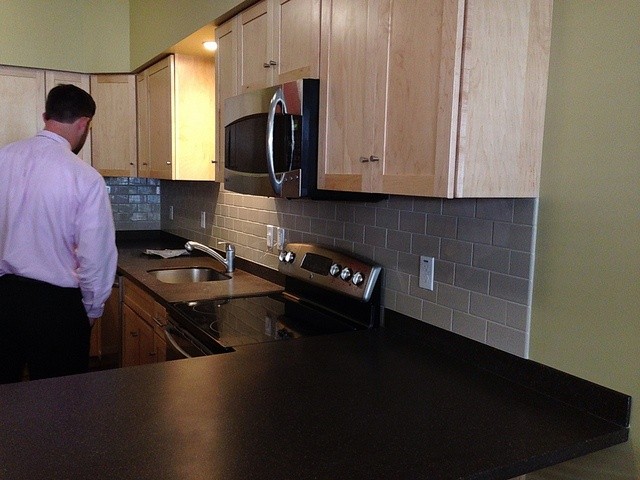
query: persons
0 83 118 385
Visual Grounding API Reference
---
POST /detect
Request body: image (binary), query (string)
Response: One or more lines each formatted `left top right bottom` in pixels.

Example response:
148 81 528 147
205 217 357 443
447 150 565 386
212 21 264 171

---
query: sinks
146 266 233 284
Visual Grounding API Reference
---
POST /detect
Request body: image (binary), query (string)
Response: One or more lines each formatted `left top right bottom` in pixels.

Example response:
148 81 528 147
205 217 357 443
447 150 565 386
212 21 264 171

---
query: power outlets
418 256 435 292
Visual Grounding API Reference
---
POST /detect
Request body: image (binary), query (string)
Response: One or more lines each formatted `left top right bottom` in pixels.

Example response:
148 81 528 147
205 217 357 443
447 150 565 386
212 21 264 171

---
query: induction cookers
166 291 365 361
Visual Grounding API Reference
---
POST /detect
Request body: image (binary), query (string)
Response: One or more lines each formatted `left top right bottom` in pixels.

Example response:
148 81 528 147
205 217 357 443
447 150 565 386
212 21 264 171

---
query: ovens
164 315 215 361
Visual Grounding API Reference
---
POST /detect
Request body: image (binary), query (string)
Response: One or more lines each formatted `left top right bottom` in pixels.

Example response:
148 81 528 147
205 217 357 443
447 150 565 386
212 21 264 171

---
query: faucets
184 240 235 273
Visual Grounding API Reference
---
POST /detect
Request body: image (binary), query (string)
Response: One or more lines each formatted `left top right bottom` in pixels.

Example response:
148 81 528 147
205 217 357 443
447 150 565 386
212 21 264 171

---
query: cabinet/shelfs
91 74 136 176
46 70 91 173
123 277 168 365
89 287 123 354
318 2 555 199
136 54 215 181
216 0 318 183
0 64 45 144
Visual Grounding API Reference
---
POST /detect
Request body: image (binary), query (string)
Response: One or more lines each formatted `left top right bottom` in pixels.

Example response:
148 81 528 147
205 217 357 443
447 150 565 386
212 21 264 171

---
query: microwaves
223 79 320 201
